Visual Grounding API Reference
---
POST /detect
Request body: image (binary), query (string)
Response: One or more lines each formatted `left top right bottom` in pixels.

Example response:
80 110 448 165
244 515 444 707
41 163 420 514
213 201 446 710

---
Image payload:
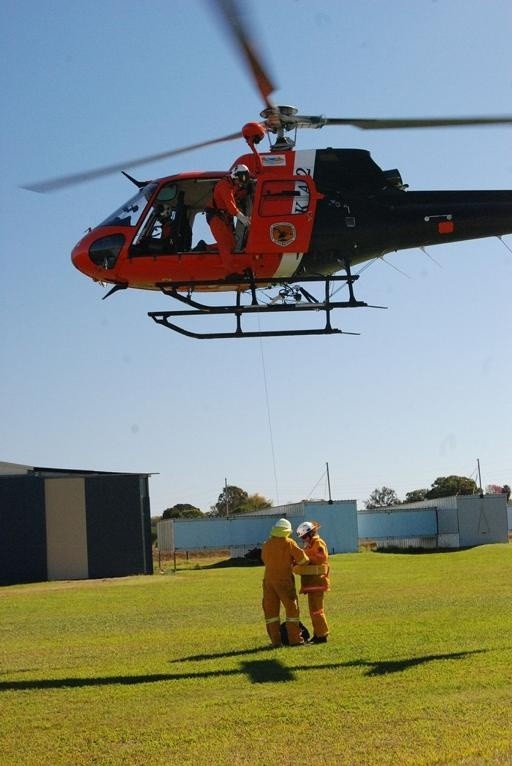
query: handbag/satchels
280 619 310 644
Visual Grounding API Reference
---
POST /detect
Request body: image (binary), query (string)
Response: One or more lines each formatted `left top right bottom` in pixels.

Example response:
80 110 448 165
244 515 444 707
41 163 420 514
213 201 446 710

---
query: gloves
236 211 253 227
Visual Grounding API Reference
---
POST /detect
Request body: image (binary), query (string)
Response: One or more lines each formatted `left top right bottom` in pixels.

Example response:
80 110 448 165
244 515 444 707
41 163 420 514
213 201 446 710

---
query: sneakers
194 239 207 252
310 634 327 645
226 272 243 280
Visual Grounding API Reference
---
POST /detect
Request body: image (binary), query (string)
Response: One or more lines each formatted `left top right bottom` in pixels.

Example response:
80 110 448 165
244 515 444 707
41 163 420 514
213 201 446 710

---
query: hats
274 516 290 531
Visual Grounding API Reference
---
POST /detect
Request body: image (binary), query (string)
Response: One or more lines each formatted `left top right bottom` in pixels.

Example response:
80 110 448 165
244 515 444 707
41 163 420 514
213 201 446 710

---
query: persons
260 517 309 646
140 204 174 256
204 164 252 254
292 521 331 643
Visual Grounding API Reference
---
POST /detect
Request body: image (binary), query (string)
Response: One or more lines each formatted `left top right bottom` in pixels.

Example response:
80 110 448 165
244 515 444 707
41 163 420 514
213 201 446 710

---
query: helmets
231 163 250 183
297 520 316 537
154 204 172 220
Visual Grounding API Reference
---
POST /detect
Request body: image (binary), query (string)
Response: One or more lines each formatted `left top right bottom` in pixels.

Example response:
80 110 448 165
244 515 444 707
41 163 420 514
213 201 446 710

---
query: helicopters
19 0 512 342
